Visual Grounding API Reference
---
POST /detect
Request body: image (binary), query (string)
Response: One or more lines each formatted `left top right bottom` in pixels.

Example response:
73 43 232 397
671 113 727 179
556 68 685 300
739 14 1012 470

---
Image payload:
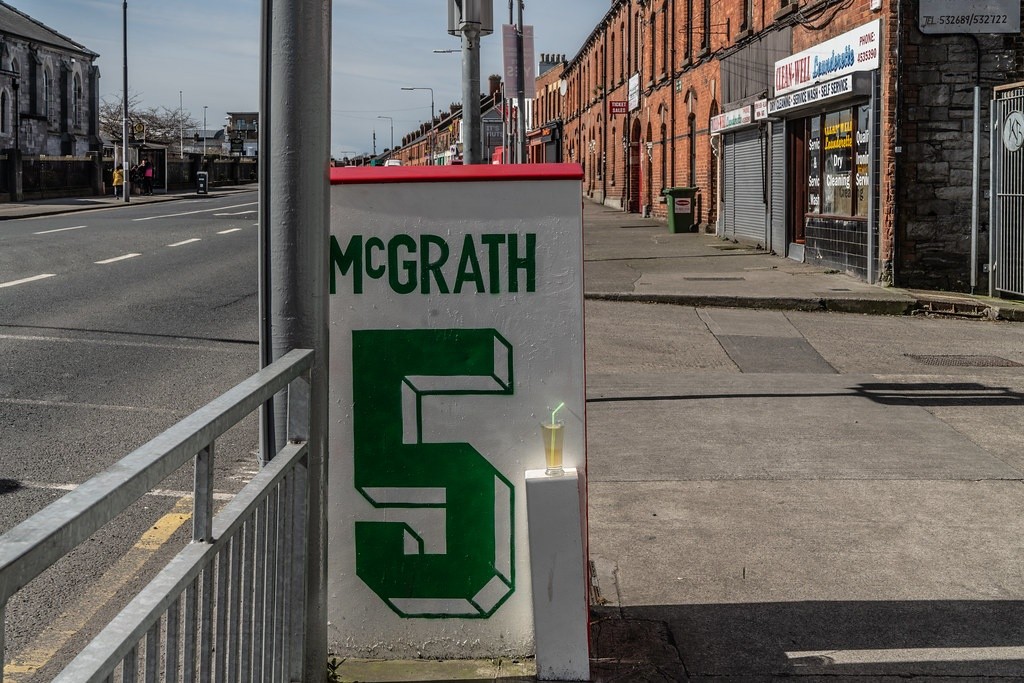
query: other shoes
115 197 119 200
149 192 153 196
144 193 150 196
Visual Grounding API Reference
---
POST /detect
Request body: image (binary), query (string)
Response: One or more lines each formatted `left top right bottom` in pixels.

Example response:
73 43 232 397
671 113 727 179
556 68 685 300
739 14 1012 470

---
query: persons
112 163 123 200
330 158 335 166
129 156 155 196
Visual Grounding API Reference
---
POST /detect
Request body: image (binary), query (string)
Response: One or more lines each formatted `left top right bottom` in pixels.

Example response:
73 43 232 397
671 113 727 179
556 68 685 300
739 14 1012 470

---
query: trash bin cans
196 172 208 194
662 186 698 233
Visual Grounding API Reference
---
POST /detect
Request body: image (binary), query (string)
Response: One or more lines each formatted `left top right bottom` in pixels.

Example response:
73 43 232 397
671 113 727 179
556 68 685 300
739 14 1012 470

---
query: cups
540 418 565 477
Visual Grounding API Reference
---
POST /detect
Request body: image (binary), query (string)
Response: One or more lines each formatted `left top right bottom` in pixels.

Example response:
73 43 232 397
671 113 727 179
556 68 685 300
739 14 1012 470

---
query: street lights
202 105 209 156
378 115 393 159
401 86 435 166
